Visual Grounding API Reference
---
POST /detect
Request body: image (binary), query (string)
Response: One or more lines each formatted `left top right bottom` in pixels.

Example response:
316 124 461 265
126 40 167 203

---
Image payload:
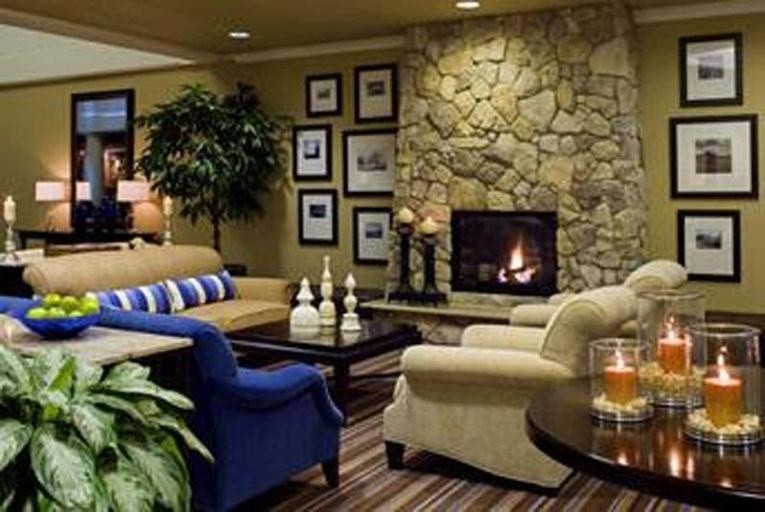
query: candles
603 350 636 401
699 352 747 431
4 193 16 225
653 324 691 379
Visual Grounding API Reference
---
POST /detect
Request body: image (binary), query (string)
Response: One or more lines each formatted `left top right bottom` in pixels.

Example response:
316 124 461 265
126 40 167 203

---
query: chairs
503 254 683 339
376 284 634 497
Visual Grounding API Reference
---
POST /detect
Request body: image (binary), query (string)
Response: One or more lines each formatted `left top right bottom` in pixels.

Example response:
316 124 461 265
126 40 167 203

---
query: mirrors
66 87 136 237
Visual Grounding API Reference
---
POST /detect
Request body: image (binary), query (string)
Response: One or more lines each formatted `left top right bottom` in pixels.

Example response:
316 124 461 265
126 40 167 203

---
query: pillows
85 279 177 316
170 269 240 310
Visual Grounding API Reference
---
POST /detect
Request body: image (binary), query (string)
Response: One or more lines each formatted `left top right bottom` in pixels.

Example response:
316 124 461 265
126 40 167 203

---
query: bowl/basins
8 300 108 339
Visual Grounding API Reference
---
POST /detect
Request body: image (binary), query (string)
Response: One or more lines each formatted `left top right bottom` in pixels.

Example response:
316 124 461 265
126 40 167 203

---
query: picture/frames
665 112 762 201
99 143 127 196
675 205 745 287
676 29 745 109
286 58 403 269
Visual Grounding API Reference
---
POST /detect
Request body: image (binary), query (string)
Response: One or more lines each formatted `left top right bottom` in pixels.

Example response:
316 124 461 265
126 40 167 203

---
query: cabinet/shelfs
13 221 158 258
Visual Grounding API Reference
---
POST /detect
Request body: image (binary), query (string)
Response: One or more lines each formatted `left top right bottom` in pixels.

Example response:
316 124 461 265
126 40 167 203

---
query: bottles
290 277 322 329
319 253 338 326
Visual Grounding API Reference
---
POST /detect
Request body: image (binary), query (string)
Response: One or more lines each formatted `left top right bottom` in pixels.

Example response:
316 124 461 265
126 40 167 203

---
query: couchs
21 234 296 335
0 289 349 512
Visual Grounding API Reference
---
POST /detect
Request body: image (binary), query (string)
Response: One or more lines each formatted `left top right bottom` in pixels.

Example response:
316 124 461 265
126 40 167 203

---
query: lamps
76 179 91 213
32 181 72 235
115 176 161 233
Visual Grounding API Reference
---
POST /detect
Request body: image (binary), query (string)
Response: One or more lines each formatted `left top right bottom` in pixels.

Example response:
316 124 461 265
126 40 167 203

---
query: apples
26 293 99 319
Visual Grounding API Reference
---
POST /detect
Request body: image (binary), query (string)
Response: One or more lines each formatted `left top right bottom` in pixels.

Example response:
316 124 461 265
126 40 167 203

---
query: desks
225 316 422 424
0 311 197 373
523 360 765 511
1 252 36 301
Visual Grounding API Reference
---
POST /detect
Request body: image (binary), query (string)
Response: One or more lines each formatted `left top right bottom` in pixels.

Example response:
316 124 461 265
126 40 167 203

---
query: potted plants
128 74 292 276
